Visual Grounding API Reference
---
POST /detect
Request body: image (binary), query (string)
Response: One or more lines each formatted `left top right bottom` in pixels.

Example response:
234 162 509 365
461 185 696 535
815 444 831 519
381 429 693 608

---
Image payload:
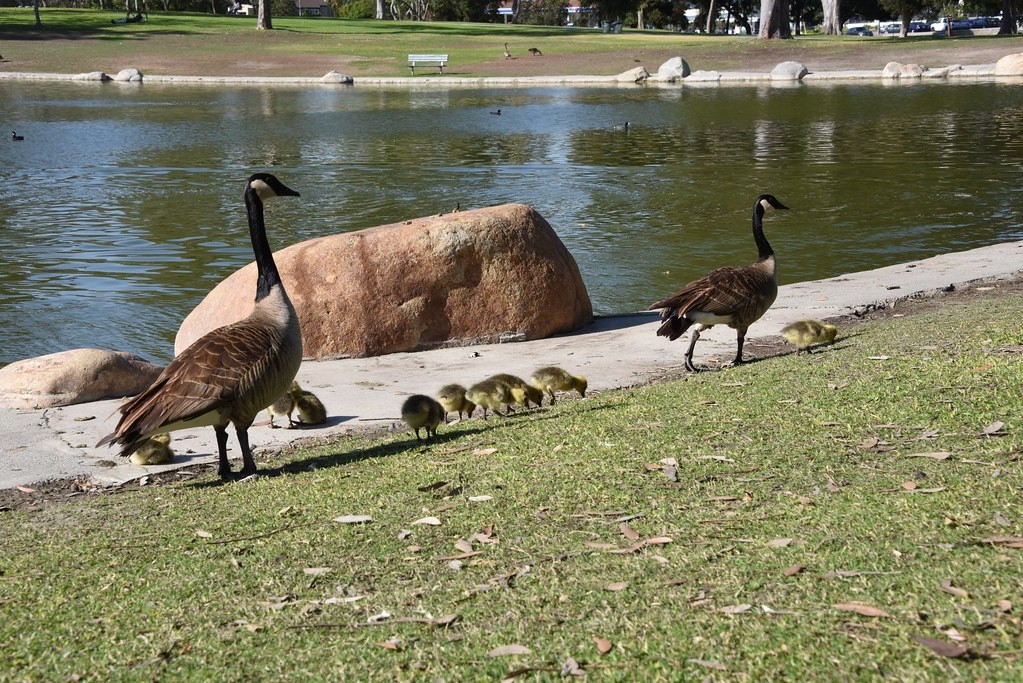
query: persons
128 13 142 22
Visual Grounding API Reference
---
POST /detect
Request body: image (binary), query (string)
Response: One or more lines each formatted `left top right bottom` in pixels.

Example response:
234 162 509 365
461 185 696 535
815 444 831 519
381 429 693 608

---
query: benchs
406 53 448 75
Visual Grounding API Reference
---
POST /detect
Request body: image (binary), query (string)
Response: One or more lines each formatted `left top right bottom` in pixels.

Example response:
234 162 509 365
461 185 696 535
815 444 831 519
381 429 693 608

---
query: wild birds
127 429 172 465
528 47 543 57
504 42 511 60
11 131 24 140
614 122 631 128
285 381 326 425
94 171 303 482
809 323 838 345
489 110 502 116
778 318 823 355
401 366 589 439
647 193 792 372
267 391 296 429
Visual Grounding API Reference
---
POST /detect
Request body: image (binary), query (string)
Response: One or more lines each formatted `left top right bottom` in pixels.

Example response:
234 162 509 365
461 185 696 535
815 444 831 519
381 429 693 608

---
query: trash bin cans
603 21 611 34
613 22 623 33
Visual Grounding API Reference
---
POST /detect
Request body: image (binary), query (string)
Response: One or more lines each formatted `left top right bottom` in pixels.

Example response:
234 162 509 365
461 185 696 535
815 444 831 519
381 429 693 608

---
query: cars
880 22 930 32
961 17 1002 29
845 27 874 36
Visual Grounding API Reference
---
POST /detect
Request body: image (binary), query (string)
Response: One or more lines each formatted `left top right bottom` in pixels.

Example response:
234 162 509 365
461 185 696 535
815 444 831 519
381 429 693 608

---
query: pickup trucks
928 17 972 32
